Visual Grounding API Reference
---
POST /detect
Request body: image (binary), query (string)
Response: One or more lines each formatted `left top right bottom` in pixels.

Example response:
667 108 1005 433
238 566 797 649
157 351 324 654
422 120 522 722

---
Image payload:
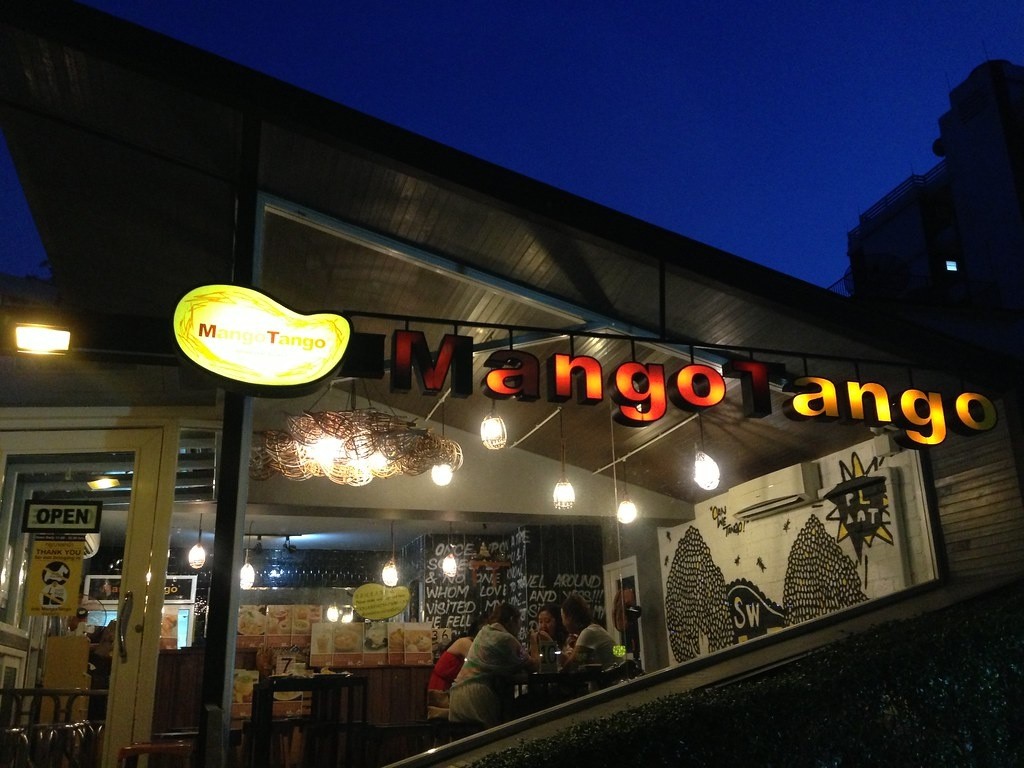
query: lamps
691 411 722 491
615 458 637 523
382 519 399 586
552 409 576 509
188 512 207 569
248 379 464 487
479 398 507 451
240 521 256 591
431 388 453 486
282 537 296 553
442 523 457 577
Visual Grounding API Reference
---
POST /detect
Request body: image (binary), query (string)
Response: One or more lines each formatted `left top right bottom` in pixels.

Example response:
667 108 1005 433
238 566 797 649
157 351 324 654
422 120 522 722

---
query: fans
50 594 107 642
612 587 642 665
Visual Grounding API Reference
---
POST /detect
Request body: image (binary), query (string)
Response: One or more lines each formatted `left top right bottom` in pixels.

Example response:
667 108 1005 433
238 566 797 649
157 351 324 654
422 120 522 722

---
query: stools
0 720 107 768
116 720 483 768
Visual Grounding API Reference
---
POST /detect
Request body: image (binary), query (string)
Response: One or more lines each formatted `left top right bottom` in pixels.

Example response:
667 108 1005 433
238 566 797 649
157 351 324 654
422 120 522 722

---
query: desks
251 677 368 768
0 689 108 768
506 672 577 713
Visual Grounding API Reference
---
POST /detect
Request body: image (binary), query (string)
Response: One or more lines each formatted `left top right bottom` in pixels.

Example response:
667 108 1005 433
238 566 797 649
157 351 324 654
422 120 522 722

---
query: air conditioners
728 462 821 524
84 533 101 560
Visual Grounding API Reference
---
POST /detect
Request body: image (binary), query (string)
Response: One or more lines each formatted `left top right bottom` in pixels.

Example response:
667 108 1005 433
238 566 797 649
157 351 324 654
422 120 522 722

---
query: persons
447 603 540 727
427 594 625 728
